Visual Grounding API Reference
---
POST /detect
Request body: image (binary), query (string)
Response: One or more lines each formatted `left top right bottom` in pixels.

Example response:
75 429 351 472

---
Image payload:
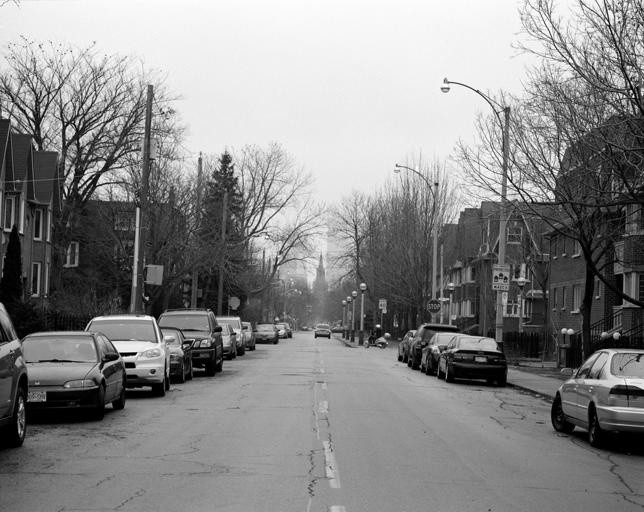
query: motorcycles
363 330 391 349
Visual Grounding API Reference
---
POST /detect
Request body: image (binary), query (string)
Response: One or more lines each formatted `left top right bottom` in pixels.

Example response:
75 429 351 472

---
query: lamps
613 332 621 340
601 331 609 340
560 327 568 344
567 328 574 344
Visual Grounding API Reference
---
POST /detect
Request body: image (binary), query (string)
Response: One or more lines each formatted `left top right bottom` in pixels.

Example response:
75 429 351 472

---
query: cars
0 302 29 449
216 315 292 360
84 314 175 396
156 308 223 376
159 327 196 383
314 323 330 339
398 323 507 387
551 348 644 447
20 330 127 421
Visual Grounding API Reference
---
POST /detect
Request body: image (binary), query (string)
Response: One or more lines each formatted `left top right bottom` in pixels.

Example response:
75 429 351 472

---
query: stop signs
427 299 442 315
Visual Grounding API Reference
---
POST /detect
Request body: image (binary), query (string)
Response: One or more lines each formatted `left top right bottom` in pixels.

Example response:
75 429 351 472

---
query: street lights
342 282 367 345
394 163 439 323
440 77 510 344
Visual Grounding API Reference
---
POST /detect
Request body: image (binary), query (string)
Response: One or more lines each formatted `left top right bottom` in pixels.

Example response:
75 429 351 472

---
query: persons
365 324 382 348
